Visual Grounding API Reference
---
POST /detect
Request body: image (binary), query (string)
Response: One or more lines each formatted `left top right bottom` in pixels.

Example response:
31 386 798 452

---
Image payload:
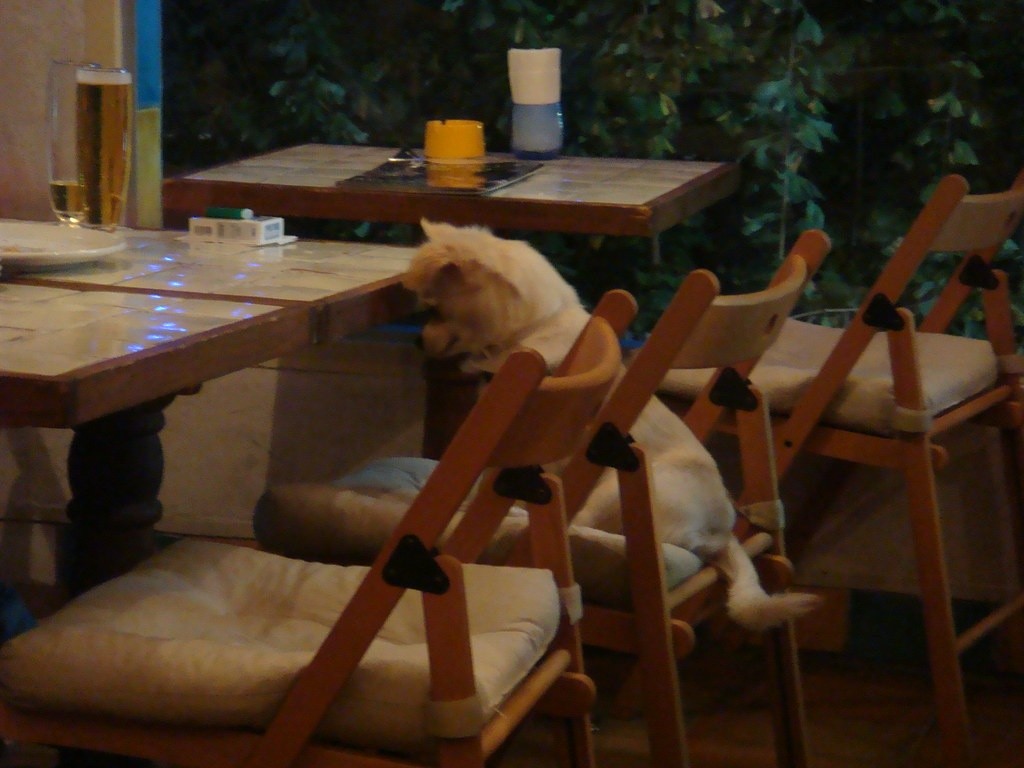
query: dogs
403 217 822 632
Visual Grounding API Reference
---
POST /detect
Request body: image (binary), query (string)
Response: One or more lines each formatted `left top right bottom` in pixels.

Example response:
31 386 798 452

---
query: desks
0 214 422 598
179 144 742 459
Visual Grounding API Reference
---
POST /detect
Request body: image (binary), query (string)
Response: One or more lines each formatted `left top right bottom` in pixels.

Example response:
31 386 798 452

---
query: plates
0 221 127 274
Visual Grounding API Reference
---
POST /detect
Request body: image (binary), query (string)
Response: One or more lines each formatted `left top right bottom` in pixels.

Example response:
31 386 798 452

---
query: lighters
207 207 254 220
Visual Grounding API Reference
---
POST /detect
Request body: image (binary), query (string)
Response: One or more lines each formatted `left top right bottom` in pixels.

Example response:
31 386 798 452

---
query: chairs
625 172 1024 768
255 225 832 768
0 289 640 768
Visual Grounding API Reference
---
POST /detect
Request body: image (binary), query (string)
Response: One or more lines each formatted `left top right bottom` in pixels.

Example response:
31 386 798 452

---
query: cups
75 66 134 228
46 57 101 222
511 103 563 159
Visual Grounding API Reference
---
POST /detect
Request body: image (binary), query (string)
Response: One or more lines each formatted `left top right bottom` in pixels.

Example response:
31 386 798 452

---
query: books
336 146 544 195
